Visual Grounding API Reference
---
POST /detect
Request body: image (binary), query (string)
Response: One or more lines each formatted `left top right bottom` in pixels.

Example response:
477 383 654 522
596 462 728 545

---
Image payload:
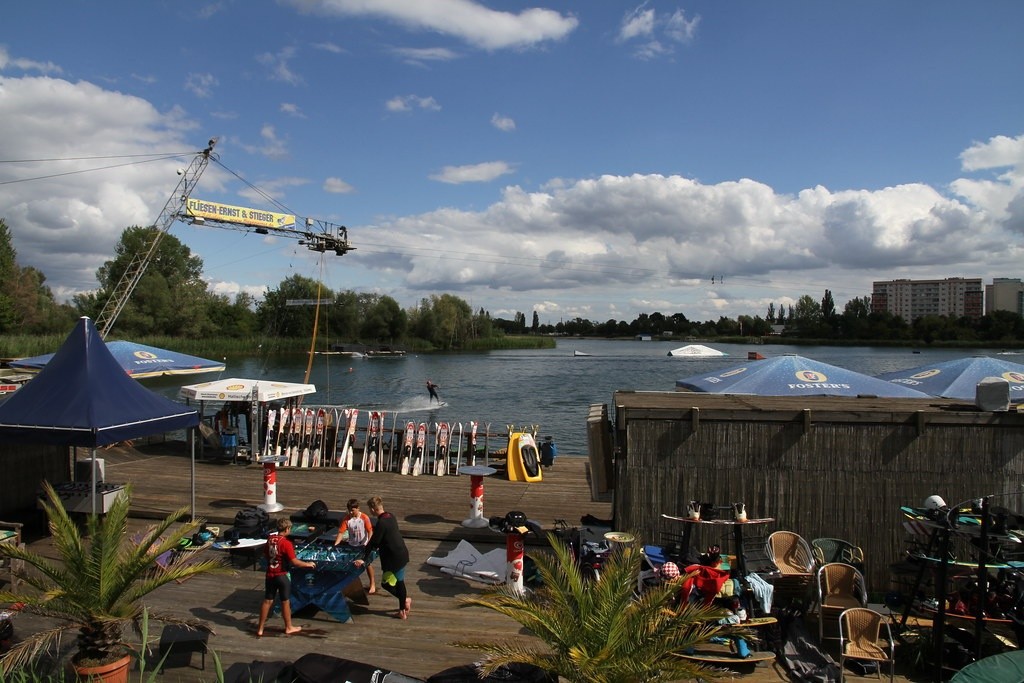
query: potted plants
0 477 239 683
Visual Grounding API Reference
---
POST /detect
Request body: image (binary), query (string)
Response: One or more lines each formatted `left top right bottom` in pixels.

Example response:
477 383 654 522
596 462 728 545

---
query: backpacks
233 505 270 528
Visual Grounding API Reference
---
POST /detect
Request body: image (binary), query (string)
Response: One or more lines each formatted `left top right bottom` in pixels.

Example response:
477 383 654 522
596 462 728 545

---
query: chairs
765 531 895 683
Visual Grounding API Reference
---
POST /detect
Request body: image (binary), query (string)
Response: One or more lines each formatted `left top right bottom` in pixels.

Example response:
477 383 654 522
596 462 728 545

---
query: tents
675 352 933 398
0 315 200 534
7 339 225 380
873 354 1024 400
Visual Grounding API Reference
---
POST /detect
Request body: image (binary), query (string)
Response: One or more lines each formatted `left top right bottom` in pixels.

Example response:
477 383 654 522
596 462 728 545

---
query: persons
426 380 441 404
334 499 375 594
257 518 316 635
353 496 411 621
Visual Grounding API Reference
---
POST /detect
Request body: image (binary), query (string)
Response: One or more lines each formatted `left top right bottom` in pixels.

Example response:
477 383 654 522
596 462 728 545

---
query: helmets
925 494 947 513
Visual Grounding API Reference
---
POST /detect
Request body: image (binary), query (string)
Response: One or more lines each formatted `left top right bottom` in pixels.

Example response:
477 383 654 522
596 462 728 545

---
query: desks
249 455 289 513
457 465 497 528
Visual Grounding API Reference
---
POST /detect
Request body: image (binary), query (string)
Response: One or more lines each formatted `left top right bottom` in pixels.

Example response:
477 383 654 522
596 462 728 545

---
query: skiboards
274 407 290 467
260 409 277 465
506 424 539 444
360 410 492 477
283 407 359 471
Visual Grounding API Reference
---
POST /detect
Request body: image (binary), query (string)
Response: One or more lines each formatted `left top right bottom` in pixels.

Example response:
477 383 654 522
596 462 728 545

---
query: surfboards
732 616 778 627
670 650 777 662
212 537 268 550
439 402 446 406
185 526 220 551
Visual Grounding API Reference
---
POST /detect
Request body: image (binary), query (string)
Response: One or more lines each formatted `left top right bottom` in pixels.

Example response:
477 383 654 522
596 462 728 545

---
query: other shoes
921 565 1024 627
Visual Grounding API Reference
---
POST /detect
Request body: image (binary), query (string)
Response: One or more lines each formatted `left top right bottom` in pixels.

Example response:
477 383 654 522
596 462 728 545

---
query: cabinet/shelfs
655 499 778 665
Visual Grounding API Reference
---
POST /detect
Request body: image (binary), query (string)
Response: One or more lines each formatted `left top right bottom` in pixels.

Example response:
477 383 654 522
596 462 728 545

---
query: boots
686 646 694 656
735 638 750 659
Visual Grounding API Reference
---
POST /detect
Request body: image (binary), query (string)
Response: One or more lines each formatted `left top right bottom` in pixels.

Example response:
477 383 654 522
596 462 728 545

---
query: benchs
224 518 278 570
160 624 209 675
290 508 348 525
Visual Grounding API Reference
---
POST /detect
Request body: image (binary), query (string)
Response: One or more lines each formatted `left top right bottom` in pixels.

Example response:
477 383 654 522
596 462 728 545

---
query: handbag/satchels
175 523 216 550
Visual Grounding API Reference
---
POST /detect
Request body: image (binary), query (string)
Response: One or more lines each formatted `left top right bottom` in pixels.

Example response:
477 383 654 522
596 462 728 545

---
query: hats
653 562 681 584
